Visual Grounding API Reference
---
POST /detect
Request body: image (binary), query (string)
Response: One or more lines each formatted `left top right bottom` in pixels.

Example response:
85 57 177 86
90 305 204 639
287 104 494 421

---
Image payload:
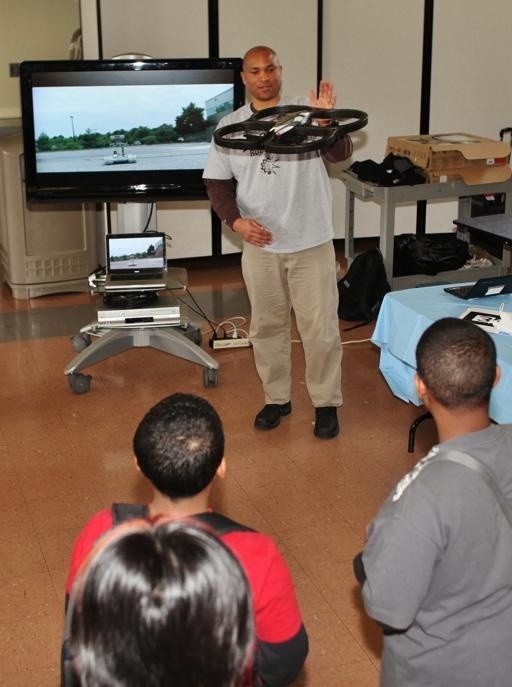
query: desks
371 283 512 453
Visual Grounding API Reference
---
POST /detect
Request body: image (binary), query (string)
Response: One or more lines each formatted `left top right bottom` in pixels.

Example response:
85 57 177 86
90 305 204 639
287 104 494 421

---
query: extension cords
210 335 251 350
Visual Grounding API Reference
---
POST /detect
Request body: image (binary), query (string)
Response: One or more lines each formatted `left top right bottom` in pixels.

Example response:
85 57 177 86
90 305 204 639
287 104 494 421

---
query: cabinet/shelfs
1 131 98 300
80 0 511 260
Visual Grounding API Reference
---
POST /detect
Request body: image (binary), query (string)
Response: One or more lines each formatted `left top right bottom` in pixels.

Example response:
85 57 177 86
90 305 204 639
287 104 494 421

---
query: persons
352 317 512 687
60 391 310 687
204 47 356 439
70 513 256 687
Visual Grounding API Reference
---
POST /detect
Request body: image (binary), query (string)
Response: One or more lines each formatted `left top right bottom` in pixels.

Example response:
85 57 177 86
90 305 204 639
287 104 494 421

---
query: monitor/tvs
18 57 249 204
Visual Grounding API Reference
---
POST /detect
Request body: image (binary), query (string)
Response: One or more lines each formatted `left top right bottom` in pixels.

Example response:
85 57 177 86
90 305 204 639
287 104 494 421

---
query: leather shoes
312 406 339 439
254 400 292 431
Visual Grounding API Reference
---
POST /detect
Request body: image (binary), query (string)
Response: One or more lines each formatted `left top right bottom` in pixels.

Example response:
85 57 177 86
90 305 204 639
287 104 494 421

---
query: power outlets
208 336 253 349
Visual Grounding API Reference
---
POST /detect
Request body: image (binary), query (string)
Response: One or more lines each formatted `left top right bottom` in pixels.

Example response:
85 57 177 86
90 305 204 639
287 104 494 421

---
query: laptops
443 274 512 300
103 231 169 289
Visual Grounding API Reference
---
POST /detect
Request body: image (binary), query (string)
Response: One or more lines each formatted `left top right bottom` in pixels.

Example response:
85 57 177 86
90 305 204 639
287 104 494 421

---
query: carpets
0 317 502 687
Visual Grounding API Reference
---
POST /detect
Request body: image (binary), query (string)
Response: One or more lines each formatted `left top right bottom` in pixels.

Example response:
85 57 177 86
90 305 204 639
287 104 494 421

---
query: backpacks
336 249 390 333
392 232 472 278
